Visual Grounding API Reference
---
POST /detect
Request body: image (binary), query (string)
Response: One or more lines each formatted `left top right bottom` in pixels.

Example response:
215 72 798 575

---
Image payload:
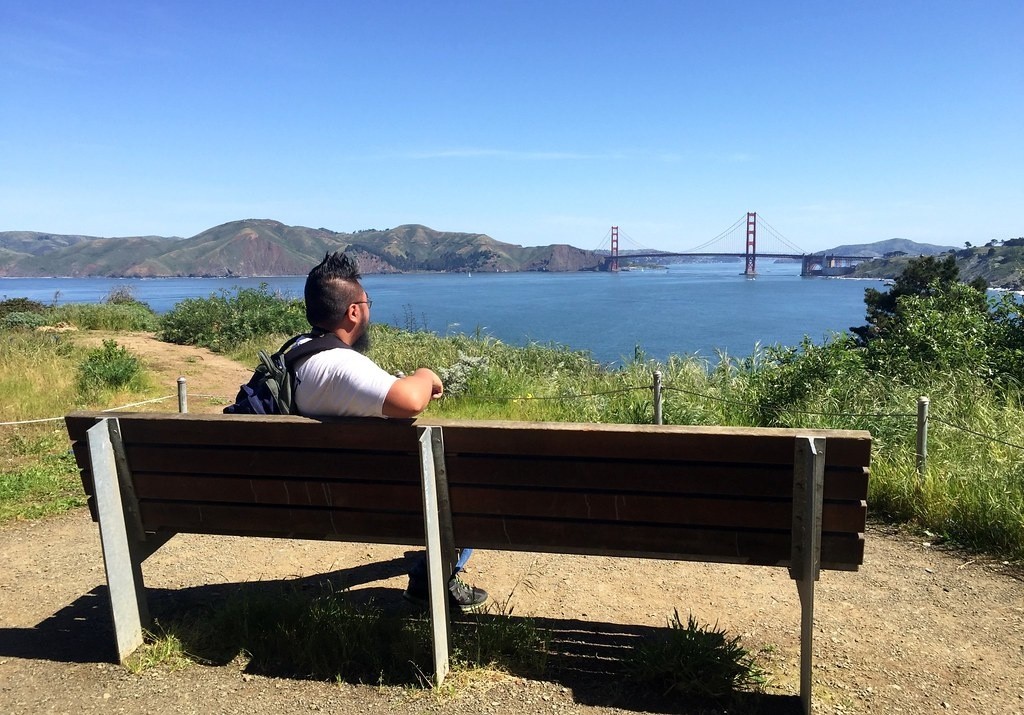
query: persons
291 251 488 611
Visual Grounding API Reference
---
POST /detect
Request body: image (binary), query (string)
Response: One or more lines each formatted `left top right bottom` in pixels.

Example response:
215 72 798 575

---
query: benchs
61 412 871 715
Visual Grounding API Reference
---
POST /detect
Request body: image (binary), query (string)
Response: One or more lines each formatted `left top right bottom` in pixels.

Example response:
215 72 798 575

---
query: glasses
344 298 373 317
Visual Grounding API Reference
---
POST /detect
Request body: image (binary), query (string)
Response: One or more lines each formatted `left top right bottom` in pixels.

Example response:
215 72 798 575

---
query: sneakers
404 576 488 611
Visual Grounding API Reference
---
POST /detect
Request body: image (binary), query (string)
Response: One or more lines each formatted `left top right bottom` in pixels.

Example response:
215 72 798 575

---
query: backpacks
223 333 355 414
